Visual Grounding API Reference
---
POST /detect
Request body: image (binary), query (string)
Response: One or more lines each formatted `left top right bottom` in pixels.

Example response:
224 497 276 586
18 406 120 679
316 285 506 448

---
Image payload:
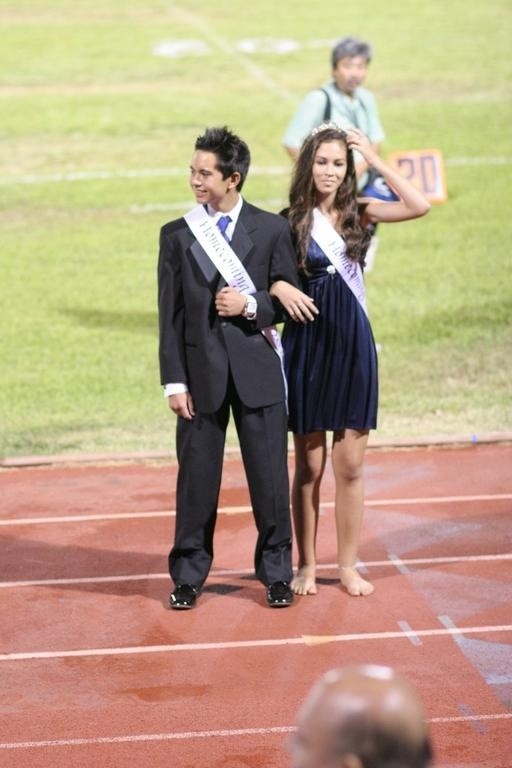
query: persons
286 664 432 768
154 124 308 608
269 120 433 600
278 37 385 279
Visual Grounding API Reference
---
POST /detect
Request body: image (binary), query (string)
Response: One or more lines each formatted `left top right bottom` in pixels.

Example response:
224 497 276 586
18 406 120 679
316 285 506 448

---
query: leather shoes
170 585 200 609
267 581 293 608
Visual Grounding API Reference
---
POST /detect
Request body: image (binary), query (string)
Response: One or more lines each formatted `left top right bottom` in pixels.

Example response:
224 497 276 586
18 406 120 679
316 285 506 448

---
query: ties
217 216 232 240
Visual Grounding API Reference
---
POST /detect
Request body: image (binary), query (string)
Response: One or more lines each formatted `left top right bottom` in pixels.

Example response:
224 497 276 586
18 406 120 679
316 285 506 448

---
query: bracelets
244 296 257 320
